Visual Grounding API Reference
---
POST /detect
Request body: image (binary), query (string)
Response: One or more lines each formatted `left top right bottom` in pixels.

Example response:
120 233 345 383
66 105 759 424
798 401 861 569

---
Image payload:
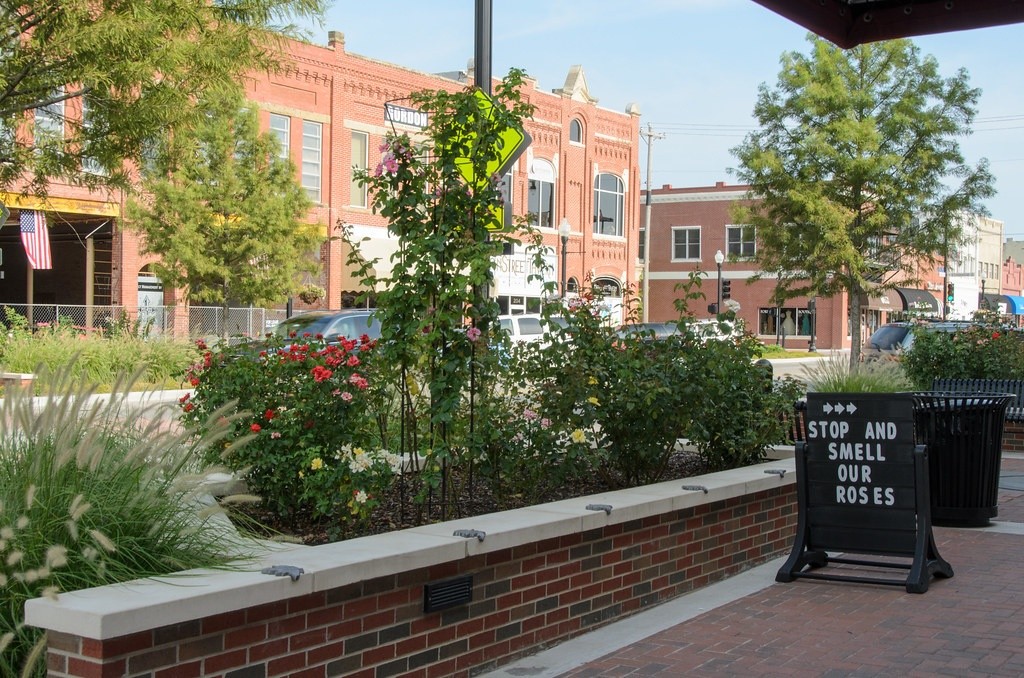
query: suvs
860 319 985 372
203 307 426 368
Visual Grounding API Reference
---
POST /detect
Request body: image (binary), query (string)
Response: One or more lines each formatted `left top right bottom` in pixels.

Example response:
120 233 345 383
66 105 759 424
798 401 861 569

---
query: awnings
860 281 1024 314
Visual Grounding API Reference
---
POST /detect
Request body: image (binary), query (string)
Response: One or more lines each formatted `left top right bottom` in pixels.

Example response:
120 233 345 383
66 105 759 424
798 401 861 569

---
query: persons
767 309 810 335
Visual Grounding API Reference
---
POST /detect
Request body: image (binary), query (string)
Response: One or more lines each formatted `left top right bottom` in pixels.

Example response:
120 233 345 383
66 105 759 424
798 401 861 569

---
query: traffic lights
721 280 731 300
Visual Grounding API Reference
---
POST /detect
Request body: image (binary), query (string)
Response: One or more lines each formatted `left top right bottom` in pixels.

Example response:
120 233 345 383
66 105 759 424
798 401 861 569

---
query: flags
20 209 53 269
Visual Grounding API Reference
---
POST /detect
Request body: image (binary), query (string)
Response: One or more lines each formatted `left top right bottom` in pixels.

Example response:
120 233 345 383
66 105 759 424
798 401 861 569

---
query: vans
488 314 590 363
611 320 739 349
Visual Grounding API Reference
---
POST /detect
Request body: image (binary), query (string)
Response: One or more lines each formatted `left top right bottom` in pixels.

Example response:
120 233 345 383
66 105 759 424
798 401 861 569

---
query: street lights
980 271 988 311
708 248 732 319
558 217 587 316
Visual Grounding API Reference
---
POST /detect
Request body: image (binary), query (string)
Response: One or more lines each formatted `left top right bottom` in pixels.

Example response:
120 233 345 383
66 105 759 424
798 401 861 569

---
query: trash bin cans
891 388 1019 528
